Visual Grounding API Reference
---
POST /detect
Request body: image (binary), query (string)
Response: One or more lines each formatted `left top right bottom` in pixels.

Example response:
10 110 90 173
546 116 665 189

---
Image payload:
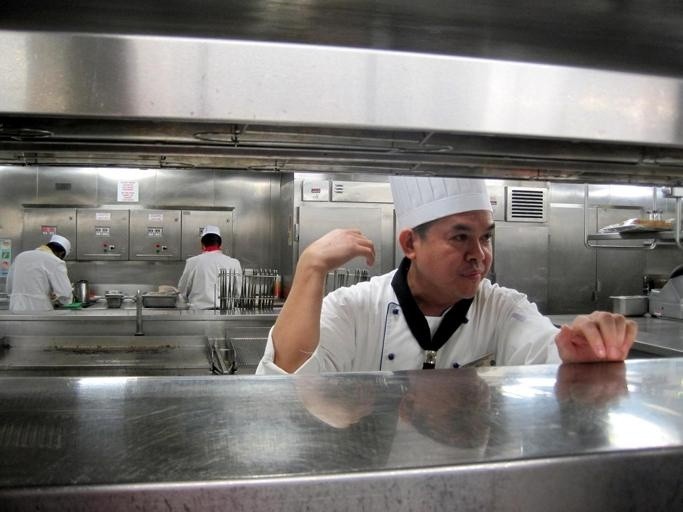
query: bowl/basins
142 294 176 307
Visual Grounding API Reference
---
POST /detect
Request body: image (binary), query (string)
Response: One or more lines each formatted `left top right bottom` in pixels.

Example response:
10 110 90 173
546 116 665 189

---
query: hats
49 234 71 260
388 174 493 232
200 225 221 240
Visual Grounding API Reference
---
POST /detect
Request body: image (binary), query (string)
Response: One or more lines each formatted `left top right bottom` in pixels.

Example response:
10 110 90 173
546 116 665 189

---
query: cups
105 289 121 308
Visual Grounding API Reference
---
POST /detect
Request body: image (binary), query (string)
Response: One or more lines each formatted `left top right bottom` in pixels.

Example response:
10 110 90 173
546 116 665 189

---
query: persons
175 233 245 309
253 209 639 377
6 234 74 315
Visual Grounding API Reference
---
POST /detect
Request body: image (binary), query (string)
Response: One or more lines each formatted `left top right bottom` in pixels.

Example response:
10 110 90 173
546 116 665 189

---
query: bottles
76 280 89 304
273 270 282 300
